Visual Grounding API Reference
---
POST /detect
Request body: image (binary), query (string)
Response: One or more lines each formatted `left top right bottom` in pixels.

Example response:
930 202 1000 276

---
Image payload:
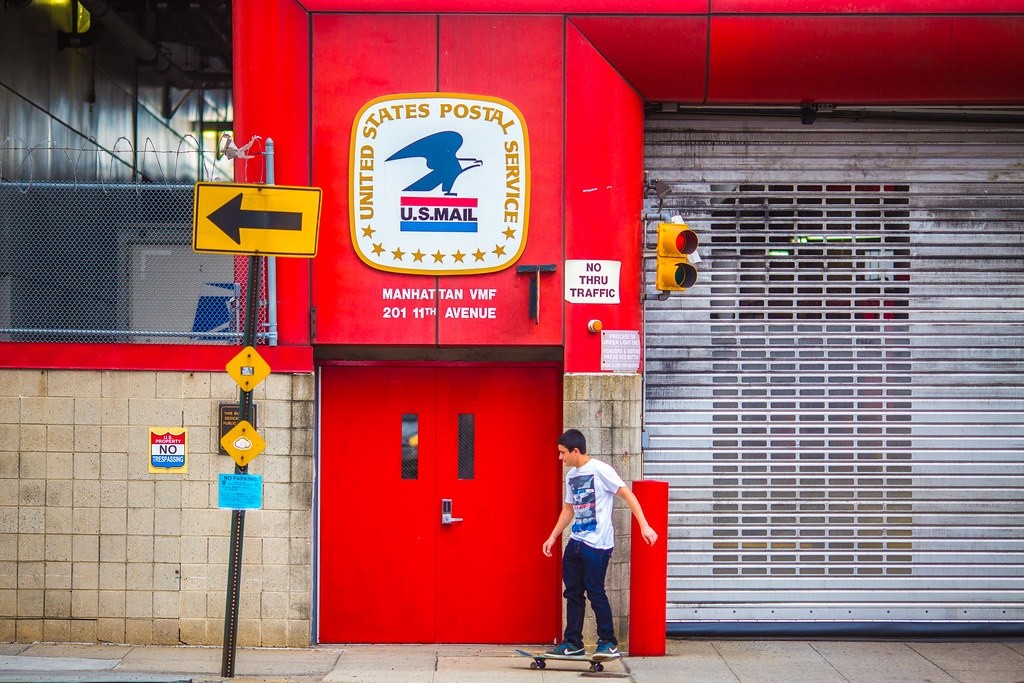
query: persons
542 429 658 662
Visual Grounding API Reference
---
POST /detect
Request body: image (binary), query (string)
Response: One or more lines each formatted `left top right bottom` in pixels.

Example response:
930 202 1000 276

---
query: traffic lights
655 219 698 294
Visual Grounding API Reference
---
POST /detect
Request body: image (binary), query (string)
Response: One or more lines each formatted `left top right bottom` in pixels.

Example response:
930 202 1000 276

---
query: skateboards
513 648 623 673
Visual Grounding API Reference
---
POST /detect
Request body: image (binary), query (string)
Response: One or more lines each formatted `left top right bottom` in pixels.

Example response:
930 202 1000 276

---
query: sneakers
591 640 621 662
544 639 586 660
